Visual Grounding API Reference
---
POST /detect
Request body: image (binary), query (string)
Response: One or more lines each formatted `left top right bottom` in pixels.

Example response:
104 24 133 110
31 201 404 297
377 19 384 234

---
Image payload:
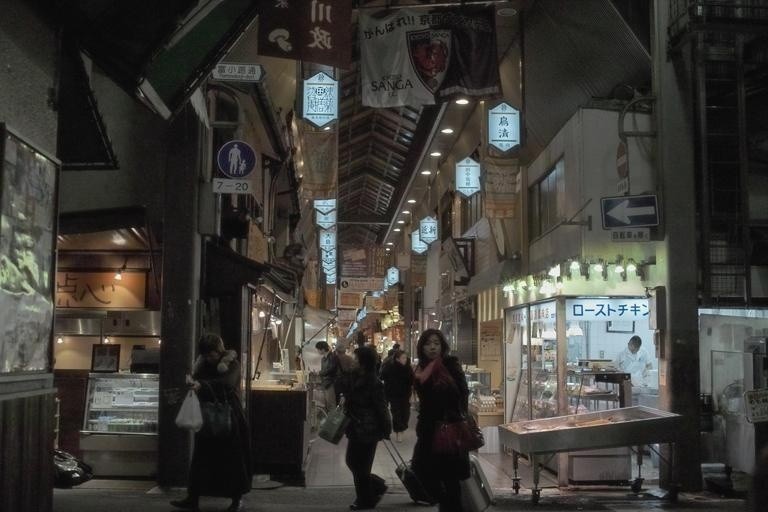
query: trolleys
500 404 696 504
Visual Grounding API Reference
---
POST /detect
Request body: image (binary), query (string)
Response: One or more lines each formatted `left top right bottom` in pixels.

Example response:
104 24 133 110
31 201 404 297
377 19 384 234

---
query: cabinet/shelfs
466 404 504 453
248 381 316 485
87 390 158 432
464 370 491 404
508 369 630 423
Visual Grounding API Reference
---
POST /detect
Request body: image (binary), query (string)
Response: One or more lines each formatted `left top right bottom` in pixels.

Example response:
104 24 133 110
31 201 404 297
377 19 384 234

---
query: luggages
458 455 498 510
381 437 438 505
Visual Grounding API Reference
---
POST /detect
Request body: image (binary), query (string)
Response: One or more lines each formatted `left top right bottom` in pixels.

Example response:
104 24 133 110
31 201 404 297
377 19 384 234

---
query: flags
354 1 504 109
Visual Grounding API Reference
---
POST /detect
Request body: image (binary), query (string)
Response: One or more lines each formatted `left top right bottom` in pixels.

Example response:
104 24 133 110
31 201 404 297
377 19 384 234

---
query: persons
406 329 471 511
337 345 393 511
314 330 414 443
615 335 652 388
168 333 254 510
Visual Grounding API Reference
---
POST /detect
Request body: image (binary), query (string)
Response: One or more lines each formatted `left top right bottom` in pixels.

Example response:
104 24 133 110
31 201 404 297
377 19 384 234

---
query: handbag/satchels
319 411 351 444
203 403 232 433
434 417 484 453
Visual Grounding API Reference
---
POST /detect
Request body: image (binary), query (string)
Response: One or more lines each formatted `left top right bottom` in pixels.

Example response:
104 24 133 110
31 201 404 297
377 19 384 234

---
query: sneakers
227 496 244 511
171 495 199 509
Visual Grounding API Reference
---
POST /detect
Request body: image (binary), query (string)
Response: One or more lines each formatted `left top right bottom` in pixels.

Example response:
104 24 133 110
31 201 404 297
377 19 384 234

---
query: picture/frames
90 342 120 372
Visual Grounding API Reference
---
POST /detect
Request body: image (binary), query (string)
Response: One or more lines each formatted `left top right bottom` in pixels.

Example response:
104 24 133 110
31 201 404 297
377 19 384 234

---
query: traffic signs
601 195 659 242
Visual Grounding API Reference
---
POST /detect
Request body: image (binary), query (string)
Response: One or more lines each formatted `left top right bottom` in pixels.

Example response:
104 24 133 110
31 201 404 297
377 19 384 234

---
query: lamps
113 267 123 282
567 320 584 338
540 316 557 341
499 253 646 298
